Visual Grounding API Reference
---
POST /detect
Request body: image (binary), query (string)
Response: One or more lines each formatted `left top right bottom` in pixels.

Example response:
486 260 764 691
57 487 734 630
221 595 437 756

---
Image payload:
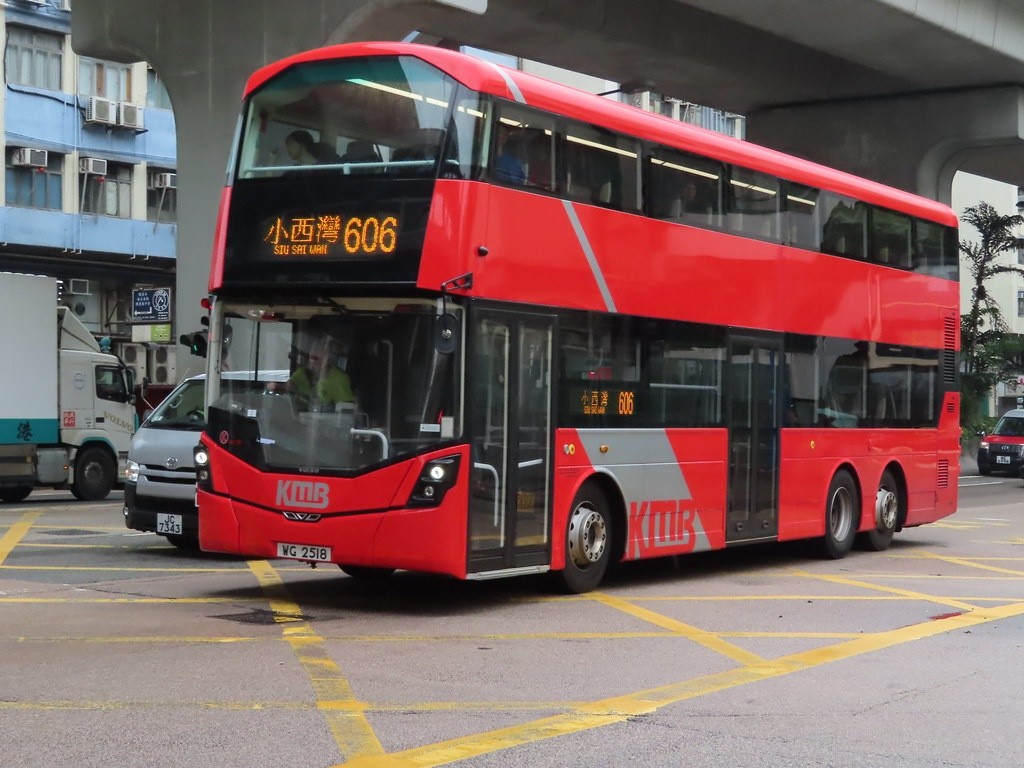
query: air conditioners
118 343 146 384
84 96 116 124
79 158 106 176
62 279 92 295
13 147 48 168
116 102 144 129
154 173 176 188
150 345 177 386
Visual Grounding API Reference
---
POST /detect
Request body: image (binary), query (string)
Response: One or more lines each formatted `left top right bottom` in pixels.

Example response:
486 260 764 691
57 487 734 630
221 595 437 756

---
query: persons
493 126 526 185
279 130 332 176
683 182 708 223
266 337 353 408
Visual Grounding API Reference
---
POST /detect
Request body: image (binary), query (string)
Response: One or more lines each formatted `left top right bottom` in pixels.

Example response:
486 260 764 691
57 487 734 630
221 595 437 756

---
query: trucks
0 271 140 502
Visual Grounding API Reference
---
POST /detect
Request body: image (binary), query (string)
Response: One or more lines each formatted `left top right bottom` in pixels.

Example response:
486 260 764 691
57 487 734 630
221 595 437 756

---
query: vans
977 408 1024 476
122 370 291 546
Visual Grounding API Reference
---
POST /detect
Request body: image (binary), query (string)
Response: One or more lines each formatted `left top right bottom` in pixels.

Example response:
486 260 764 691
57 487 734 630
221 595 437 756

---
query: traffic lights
179 328 230 359
198 295 225 328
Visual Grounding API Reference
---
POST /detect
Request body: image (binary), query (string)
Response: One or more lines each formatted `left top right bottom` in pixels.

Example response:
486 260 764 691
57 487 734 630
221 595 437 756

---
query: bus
192 41 962 592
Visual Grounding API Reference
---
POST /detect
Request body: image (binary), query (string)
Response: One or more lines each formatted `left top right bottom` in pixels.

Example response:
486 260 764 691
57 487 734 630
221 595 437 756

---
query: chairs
317 138 942 273
473 354 924 449
